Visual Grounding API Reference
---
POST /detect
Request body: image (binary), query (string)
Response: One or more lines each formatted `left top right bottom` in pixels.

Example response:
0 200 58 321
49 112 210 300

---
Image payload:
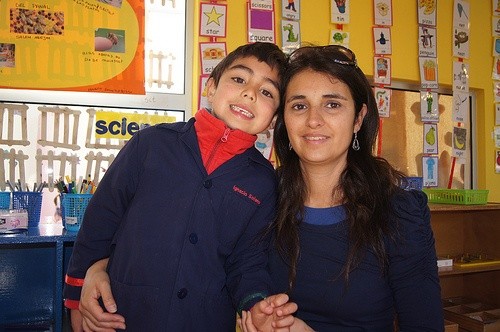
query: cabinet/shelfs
0 226 76 332
426 201 500 332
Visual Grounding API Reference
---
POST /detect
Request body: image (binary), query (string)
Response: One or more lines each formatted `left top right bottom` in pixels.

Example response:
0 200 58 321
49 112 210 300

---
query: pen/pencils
6 179 46 192
54 173 97 194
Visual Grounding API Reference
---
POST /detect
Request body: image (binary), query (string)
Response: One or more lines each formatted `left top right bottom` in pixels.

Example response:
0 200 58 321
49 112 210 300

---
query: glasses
286 44 358 71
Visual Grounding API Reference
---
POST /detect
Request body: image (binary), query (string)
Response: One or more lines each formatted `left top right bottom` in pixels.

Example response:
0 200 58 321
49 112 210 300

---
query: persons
78 41 444 332
63 42 297 332
